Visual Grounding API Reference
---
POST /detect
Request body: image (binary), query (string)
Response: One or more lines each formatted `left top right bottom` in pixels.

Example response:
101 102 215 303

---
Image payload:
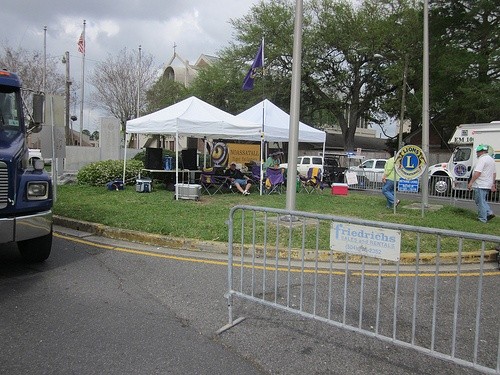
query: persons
467 145 497 224
380 149 400 209
225 161 253 196
263 150 284 177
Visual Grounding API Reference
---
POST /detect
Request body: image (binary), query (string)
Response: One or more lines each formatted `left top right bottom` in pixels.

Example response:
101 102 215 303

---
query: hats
268 150 284 156
476 144 488 151
231 162 236 166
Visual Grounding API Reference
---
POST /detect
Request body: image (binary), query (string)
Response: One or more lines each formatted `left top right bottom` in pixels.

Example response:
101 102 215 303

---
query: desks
142 168 202 190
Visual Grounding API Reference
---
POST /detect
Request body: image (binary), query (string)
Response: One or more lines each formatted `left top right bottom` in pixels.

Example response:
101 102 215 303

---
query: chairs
201 167 323 196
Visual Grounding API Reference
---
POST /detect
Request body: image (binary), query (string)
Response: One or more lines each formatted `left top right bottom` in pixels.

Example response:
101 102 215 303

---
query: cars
342 158 389 188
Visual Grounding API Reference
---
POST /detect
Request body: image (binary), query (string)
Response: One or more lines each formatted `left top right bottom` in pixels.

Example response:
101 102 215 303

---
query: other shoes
395 199 400 207
243 191 250 195
487 213 495 221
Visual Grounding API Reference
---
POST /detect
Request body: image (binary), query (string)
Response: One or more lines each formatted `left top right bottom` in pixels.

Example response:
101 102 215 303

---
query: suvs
279 155 339 185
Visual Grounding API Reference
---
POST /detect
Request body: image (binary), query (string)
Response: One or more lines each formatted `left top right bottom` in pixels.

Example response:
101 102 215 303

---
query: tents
204 98 326 181
123 95 264 200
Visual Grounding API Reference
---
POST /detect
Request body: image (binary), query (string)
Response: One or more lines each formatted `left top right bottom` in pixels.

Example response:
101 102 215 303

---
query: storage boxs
331 183 348 195
136 179 151 192
174 183 202 200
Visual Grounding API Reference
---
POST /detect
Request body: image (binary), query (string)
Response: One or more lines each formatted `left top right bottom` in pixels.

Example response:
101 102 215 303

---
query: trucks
0 68 55 262
427 121 500 202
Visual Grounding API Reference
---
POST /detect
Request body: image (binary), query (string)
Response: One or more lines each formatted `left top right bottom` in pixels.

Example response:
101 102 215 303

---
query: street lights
373 52 410 154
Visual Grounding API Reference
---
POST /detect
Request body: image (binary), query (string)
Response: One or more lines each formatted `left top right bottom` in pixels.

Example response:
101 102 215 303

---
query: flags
77 28 85 54
241 44 262 90
